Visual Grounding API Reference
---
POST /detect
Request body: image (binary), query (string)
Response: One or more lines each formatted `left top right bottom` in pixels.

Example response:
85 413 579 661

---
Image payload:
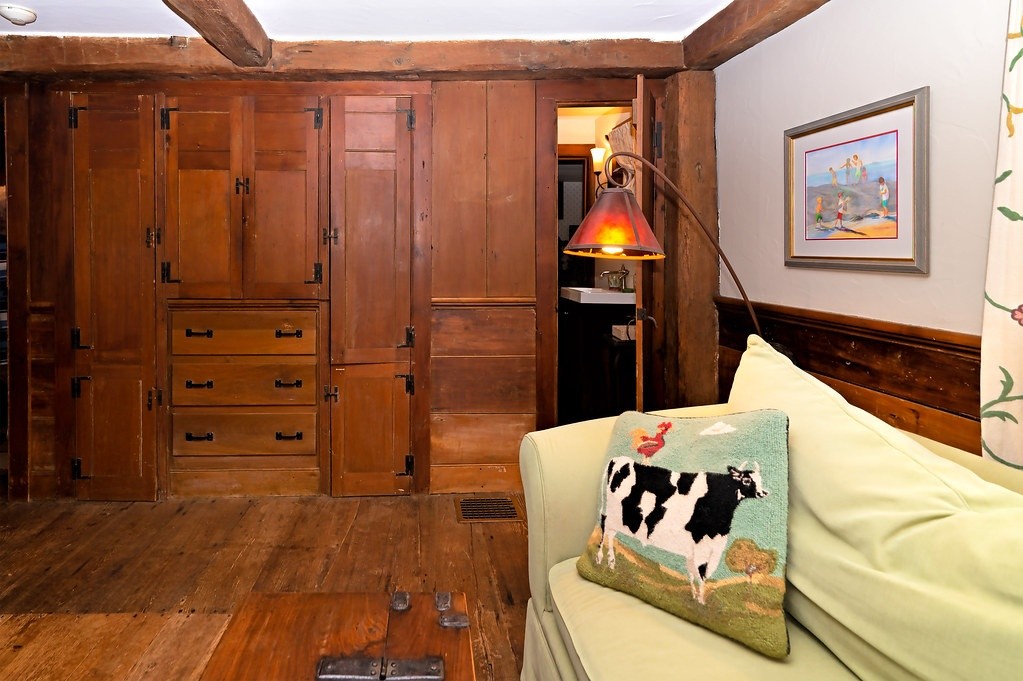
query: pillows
576 407 790 658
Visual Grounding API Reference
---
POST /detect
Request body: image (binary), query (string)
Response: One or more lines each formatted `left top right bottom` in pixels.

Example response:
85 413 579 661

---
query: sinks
560 287 636 304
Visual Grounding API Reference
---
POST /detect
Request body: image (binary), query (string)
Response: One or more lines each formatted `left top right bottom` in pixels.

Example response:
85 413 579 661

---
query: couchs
518 334 1023 681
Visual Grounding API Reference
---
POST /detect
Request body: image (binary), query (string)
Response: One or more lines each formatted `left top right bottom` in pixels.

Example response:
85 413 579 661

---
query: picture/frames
783 86 929 275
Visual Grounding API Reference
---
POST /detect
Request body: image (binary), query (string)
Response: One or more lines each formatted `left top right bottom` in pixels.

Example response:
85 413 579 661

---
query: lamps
562 150 760 335
590 148 607 198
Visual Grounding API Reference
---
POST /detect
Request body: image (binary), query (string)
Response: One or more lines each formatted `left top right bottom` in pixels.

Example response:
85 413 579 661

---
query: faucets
600 264 630 290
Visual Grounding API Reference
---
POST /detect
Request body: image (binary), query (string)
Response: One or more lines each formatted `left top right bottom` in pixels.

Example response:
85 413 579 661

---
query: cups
607 274 622 287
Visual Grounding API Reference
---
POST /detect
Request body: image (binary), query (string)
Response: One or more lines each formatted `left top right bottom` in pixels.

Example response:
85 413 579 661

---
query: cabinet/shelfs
0 76 558 504
